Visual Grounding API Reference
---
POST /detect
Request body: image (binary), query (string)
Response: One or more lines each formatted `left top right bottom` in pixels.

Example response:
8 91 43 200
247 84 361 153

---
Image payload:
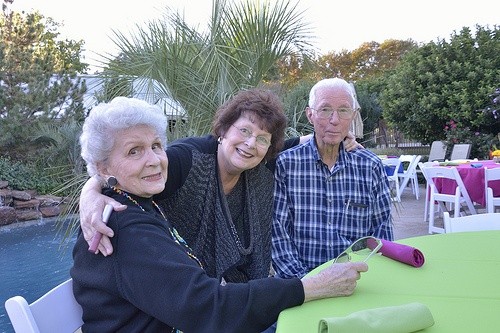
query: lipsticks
88 204 113 253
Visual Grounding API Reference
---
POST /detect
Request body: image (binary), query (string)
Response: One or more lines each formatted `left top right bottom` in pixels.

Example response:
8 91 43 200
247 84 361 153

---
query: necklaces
215 167 253 254
113 187 202 266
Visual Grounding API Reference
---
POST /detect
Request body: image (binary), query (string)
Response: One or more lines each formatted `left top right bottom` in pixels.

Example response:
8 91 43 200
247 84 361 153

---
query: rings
89 235 93 240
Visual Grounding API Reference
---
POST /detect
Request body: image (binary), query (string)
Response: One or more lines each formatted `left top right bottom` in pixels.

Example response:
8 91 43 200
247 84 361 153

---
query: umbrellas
349 83 364 138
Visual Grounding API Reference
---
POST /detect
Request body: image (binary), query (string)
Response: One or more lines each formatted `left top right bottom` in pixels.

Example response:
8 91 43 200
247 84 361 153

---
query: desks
276 230 500 333
382 159 404 203
427 159 500 228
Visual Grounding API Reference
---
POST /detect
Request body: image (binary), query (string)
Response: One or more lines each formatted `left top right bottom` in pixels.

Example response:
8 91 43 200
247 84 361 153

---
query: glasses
312 107 359 118
333 236 383 265
230 123 273 149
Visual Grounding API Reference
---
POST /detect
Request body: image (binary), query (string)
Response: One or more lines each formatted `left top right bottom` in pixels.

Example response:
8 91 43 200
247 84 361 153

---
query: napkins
365 237 425 268
471 163 483 168
318 304 435 333
439 162 458 166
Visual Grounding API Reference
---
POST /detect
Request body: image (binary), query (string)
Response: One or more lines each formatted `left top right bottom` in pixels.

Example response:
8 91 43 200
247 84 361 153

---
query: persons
78 91 364 283
269 78 394 280
69 97 368 333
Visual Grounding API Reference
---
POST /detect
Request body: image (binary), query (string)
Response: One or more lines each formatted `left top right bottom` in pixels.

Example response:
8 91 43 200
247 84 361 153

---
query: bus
0 73 190 133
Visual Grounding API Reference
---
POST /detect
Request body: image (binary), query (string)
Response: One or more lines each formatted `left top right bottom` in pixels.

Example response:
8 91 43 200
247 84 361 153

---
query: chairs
5 278 84 333
376 141 500 234
